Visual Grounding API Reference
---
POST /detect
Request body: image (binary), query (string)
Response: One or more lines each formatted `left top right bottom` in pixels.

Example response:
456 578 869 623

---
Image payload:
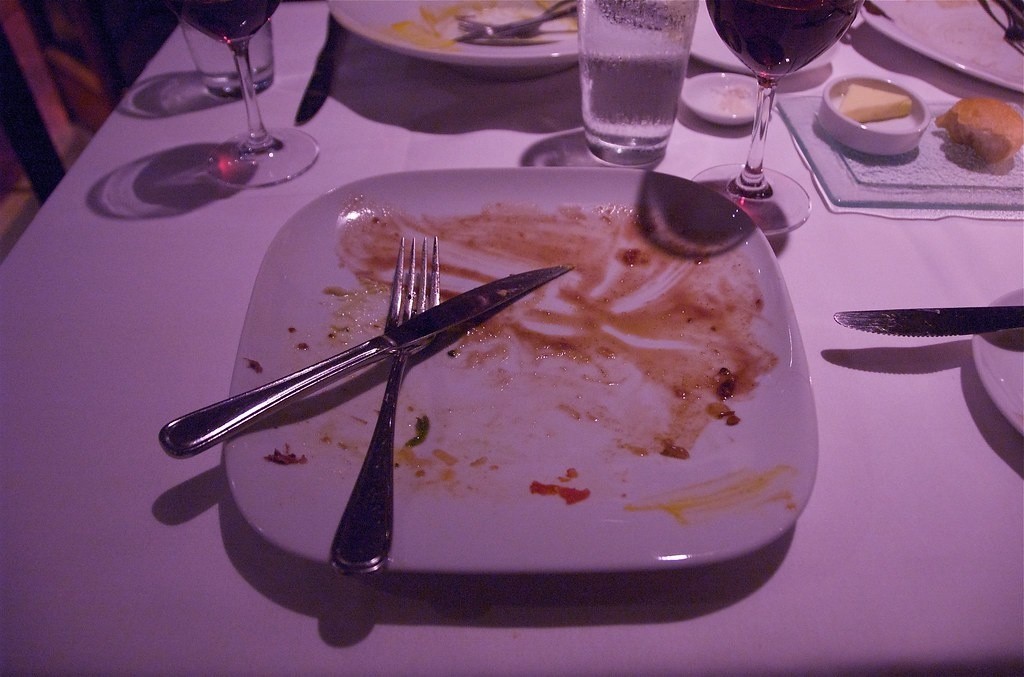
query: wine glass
688 0 860 238
176 0 321 188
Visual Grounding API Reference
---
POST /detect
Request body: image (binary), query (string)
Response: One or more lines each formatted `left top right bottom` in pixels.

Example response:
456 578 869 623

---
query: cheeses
838 83 912 123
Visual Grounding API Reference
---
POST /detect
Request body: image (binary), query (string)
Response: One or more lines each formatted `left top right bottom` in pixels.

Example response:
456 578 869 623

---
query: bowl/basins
816 74 932 155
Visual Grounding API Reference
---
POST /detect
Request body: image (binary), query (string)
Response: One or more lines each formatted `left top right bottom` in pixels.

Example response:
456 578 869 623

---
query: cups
576 0 700 169
180 16 275 99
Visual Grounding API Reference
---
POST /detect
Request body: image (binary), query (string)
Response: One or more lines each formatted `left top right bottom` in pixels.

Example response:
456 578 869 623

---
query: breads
935 96 1024 164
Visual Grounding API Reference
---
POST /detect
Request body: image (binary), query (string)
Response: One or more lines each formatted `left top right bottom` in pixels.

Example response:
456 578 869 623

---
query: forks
329 236 442 574
456 0 574 34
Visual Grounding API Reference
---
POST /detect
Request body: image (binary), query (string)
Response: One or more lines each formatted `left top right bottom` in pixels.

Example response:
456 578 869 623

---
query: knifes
158 263 576 459
834 303 1024 337
294 12 342 126
451 7 576 43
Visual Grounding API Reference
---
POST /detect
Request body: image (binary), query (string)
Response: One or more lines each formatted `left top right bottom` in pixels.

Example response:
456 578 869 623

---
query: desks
0 0 1024 677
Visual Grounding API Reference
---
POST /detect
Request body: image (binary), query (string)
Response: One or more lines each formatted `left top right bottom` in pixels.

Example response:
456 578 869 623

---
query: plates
221 167 820 573
775 94 1024 222
681 73 762 127
972 287 1024 436
689 0 840 75
329 0 579 75
862 0 1024 93
